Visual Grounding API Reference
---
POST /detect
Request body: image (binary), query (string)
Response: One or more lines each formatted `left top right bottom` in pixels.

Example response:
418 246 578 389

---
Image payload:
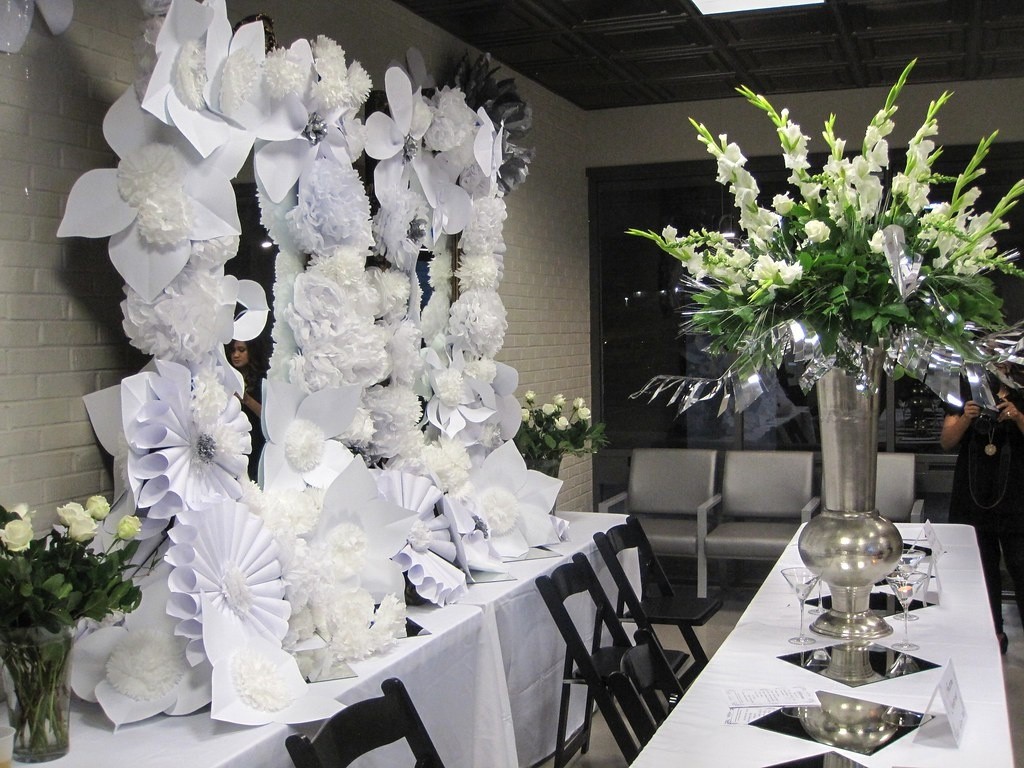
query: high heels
1000 633 1008 654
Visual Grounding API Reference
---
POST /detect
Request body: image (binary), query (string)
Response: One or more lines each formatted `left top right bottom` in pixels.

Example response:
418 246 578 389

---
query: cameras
981 405 999 417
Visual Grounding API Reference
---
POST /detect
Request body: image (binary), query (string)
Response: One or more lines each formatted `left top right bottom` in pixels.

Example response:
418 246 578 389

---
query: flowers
513 390 611 480
0 496 149 748
623 58 1024 385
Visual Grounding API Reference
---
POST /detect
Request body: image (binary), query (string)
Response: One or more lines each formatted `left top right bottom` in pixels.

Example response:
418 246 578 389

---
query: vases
523 456 564 517
1 621 79 764
795 355 904 638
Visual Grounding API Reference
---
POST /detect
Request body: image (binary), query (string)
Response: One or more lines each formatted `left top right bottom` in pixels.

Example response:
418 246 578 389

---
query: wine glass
894 548 926 621
780 645 824 718
781 567 820 645
886 572 929 651
808 578 828 615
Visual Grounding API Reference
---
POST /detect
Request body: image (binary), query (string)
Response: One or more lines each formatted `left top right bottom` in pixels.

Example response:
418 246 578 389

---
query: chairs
536 552 691 768
593 514 723 701
602 627 685 747
597 449 925 598
285 676 445 768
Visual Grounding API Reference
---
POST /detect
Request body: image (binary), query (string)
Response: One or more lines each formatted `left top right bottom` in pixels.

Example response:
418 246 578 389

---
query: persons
940 307 1024 655
229 337 271 480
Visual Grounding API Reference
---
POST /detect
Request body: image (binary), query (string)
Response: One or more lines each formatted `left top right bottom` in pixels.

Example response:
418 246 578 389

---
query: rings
1007 412 1010 417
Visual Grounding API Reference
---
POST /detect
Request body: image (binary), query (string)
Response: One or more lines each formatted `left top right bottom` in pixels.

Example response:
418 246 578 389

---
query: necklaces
985 428 997 455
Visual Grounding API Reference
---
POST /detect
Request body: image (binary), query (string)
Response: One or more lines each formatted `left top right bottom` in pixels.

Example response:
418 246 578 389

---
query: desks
0 605 510 768
628 522 1015 768
460 511 642 768
